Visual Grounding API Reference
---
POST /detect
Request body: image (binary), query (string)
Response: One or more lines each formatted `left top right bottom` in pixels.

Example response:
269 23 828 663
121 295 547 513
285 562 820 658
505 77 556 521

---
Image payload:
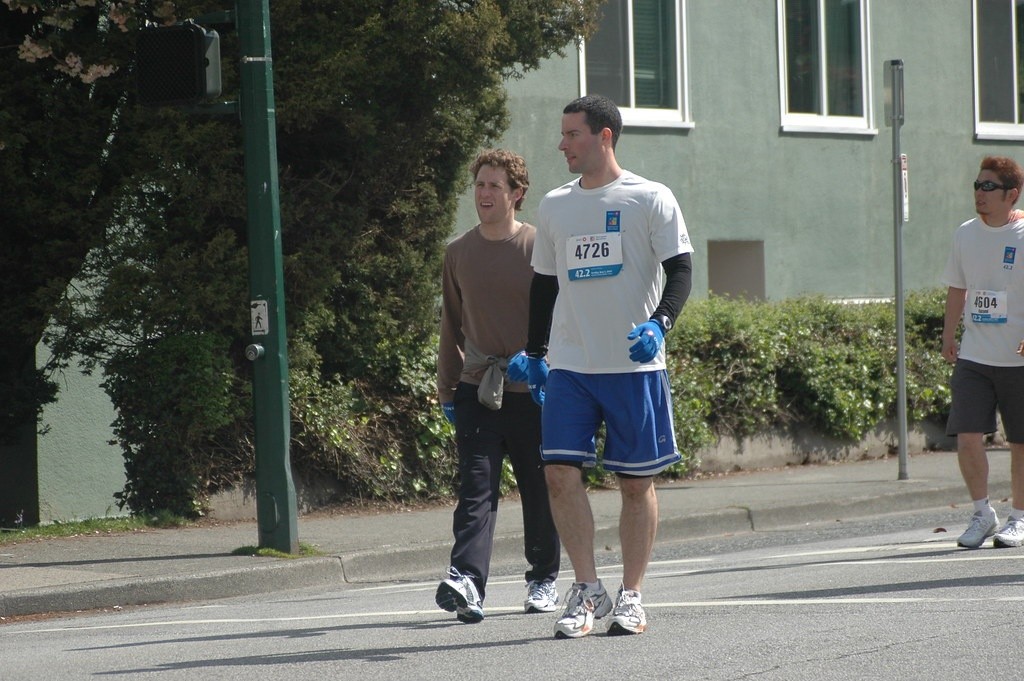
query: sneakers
553 578 614 638
993 515 1024 547
524 579 559 613
605 583 647 635
957 507 999 549
435 566 484 623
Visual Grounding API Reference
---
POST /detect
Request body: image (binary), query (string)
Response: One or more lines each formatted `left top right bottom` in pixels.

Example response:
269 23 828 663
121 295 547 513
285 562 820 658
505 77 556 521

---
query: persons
941 156 1024 550
522 92 694 638
434 147 562 622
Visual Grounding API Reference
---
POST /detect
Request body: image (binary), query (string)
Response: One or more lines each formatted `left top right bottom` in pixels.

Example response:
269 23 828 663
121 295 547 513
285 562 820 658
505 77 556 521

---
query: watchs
651 312 673 333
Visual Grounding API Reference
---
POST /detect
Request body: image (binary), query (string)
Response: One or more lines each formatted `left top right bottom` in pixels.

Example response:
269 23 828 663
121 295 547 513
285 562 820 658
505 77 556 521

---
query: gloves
527 356 549 407
505 349 528 383
627 320 666 363
441 400 457 426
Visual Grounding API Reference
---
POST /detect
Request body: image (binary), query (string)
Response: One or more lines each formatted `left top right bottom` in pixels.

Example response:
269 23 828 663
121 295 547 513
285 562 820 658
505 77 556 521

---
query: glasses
974 181 1015 192
